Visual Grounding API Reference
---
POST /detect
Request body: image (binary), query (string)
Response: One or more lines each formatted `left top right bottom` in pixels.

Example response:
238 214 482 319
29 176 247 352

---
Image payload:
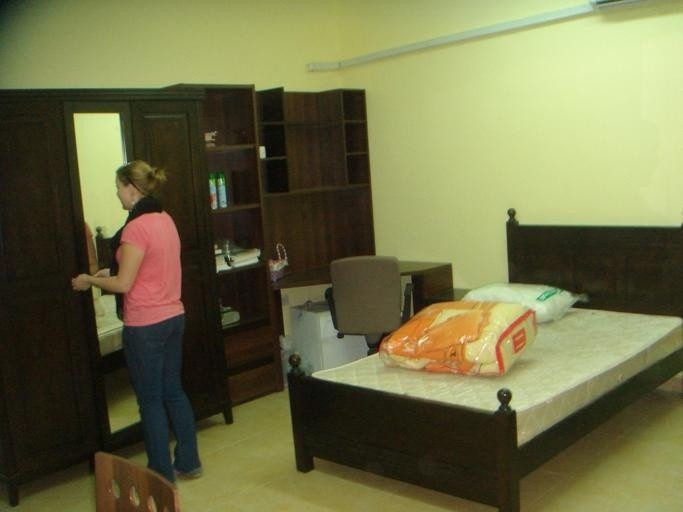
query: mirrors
61 99 143 452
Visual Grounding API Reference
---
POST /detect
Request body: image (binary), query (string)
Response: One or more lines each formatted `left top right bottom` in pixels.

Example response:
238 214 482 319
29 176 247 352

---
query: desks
259 254 454 317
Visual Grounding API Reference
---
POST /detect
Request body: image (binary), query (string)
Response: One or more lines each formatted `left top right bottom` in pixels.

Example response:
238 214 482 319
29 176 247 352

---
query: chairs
92 449 182 512
324 254 413 356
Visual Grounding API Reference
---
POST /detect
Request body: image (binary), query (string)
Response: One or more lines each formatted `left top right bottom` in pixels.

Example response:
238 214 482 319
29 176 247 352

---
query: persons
71 160 204 487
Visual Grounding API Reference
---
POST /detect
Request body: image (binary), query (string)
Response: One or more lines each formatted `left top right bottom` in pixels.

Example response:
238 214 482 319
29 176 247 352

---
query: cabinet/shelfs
256 87 372 202
165 84 284 406
289 301 369 373
1 88 108 507
126 87 236 425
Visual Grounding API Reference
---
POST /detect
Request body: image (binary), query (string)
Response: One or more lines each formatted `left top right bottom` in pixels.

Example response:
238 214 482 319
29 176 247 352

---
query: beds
284 207 681 511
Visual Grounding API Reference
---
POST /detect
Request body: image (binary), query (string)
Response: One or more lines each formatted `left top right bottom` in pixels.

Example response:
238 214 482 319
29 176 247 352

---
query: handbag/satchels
268 243 288 282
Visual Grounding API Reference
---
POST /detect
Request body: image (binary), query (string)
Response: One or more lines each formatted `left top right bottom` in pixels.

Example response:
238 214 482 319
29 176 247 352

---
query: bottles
208 173 218 211
217 172 228 208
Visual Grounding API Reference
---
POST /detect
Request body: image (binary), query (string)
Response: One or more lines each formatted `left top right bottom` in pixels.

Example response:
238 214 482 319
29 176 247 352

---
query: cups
205 132 216 147
259 146 266 159
